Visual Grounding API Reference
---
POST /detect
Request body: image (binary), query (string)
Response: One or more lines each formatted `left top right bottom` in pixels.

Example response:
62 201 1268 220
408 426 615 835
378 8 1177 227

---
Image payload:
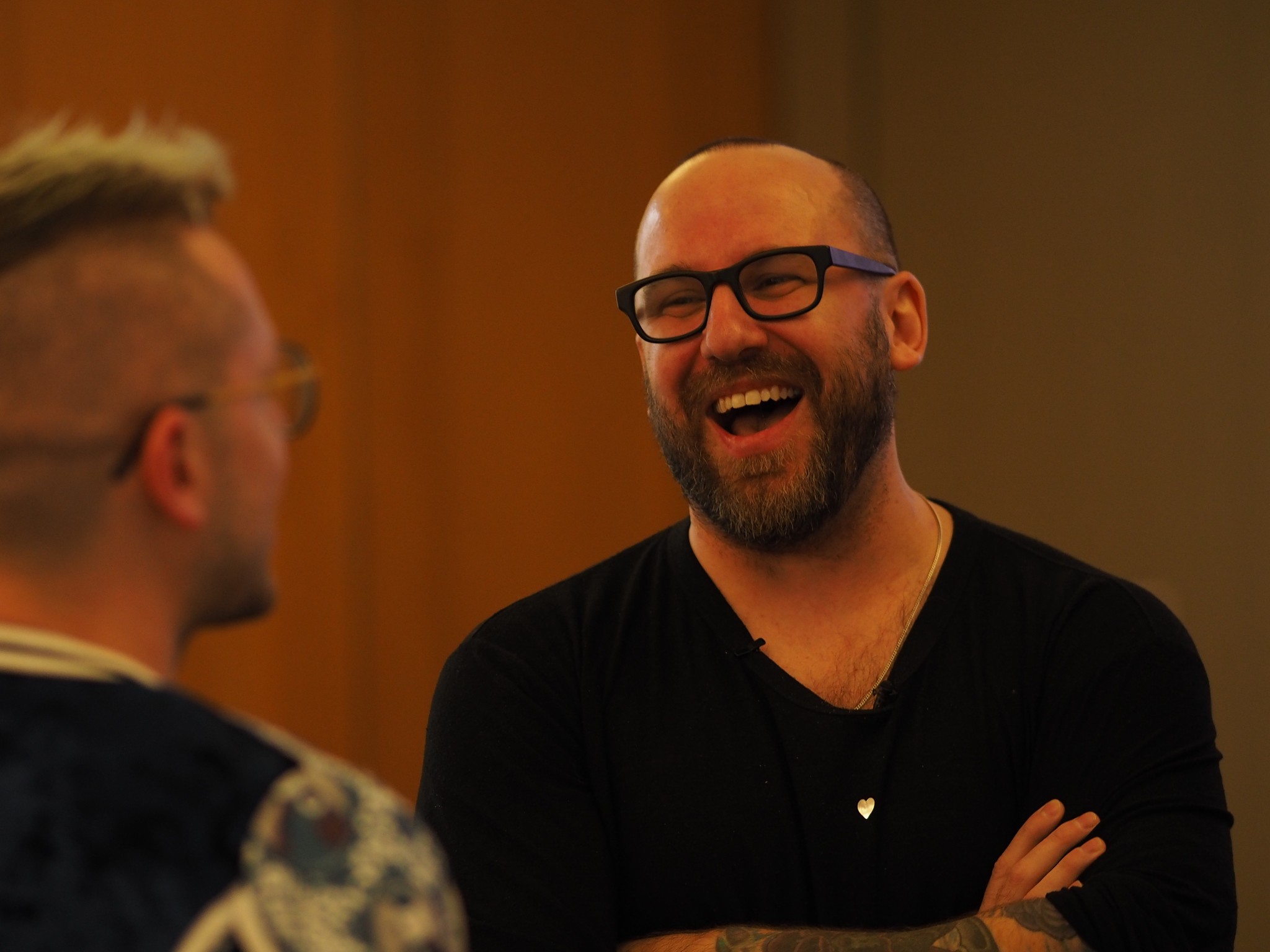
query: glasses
111 340 322 482
615 244 896 343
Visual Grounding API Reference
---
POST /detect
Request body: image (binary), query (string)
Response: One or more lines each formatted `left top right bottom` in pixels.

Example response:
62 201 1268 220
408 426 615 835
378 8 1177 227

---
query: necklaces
854 496 942 709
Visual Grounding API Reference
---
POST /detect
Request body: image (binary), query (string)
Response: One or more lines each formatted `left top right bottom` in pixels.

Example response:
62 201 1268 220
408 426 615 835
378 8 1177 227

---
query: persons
0 105 462 952
414 137 1239 952
